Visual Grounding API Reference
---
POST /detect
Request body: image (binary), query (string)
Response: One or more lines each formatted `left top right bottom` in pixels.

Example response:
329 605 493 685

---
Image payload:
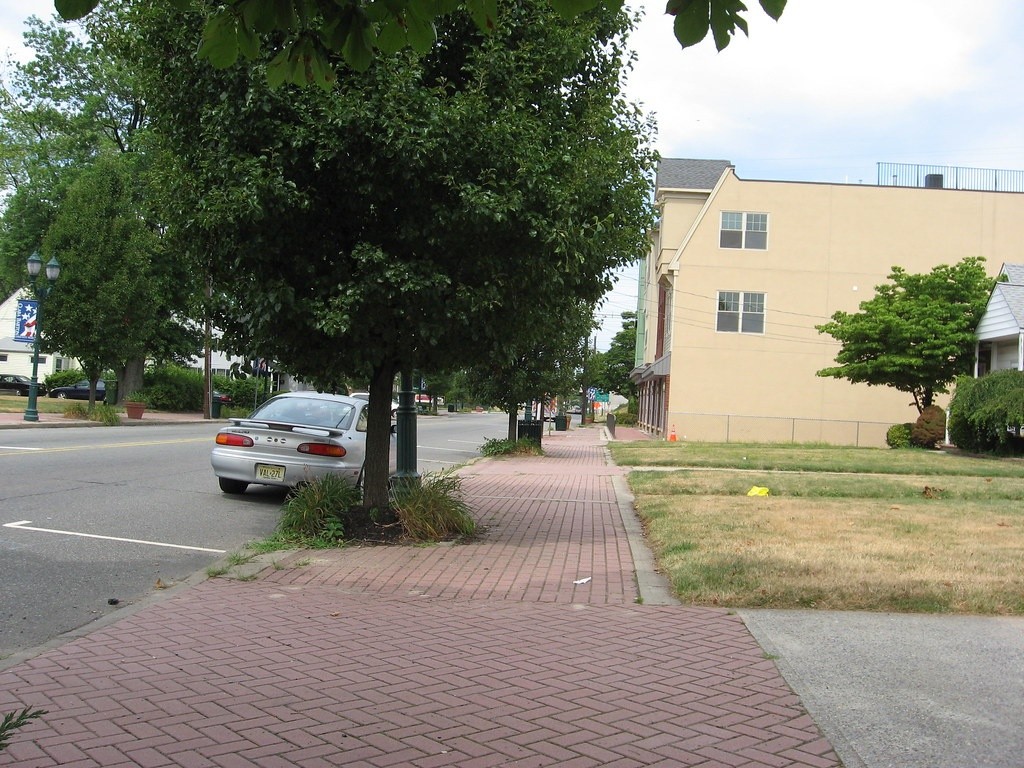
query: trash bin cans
555 415 567 431
518 420 542 448
448 404 454 412
212 396 222 418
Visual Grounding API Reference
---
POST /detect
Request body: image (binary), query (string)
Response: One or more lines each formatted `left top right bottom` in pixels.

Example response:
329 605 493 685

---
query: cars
48 378 106 401
0 374 47 396
349 392 398 433
210 392 366 506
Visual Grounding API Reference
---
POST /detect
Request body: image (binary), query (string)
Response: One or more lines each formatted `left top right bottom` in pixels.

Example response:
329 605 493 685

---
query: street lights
23 251 60 421
